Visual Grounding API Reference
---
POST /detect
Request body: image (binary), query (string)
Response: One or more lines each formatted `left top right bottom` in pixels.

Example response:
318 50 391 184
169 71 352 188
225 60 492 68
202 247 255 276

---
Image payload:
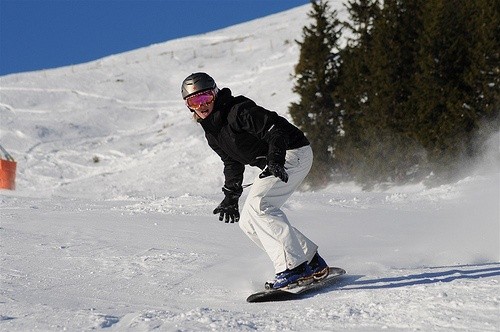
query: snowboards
246 267 346 303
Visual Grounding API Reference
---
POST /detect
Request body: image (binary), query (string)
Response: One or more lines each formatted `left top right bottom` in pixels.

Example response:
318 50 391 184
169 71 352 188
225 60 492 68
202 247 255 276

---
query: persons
181 70 329 291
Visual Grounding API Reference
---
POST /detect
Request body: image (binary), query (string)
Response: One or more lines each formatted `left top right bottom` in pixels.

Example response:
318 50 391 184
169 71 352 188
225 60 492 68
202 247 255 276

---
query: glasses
186 90 215 109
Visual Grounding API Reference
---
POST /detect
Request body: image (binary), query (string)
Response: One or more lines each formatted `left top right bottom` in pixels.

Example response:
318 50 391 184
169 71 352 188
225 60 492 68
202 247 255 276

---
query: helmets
181 72 216 99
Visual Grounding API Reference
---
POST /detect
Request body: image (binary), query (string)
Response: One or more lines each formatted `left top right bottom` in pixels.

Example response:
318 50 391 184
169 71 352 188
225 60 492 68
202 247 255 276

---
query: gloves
256 153 288 183
213 186 243 223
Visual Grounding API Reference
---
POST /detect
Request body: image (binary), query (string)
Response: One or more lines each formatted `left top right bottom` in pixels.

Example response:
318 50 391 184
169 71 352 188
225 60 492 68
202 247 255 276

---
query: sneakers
308 253 329 280
272 261 314 289
